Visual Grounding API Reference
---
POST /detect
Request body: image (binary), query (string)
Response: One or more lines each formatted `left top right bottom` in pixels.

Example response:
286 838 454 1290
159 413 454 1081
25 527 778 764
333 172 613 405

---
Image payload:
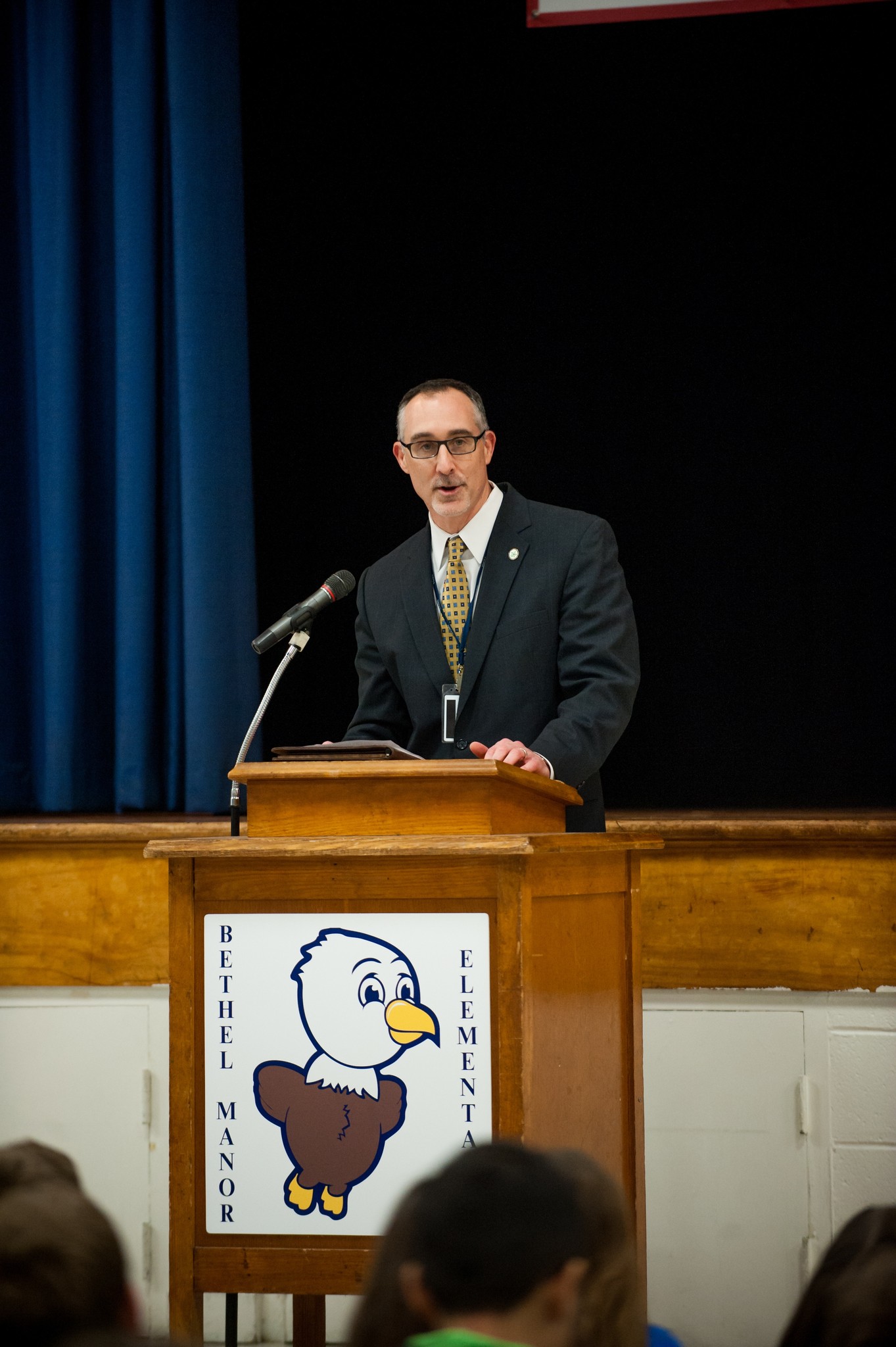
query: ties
440 537 472 694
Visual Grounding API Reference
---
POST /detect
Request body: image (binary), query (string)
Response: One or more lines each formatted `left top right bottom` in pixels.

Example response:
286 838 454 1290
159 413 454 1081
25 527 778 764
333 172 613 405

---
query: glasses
399 430 485 459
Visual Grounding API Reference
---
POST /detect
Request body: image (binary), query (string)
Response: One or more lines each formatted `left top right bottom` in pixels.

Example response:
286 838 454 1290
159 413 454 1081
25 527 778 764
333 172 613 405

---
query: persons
306 378 641 831
0 1136 896 1347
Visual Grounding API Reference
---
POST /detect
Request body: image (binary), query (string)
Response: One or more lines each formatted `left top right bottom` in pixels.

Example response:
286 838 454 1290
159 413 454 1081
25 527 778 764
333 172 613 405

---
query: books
271 745 420 761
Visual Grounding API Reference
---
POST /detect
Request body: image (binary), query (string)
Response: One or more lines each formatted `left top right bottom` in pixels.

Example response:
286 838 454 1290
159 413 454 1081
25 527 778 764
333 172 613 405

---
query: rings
520 748 527 761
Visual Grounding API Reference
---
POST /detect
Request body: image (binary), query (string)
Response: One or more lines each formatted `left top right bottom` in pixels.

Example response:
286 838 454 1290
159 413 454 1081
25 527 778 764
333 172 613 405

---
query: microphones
251 570 356 654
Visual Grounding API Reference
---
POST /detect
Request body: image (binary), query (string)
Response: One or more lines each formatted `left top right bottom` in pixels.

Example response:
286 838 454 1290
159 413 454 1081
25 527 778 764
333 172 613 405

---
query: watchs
538 754 549 766
